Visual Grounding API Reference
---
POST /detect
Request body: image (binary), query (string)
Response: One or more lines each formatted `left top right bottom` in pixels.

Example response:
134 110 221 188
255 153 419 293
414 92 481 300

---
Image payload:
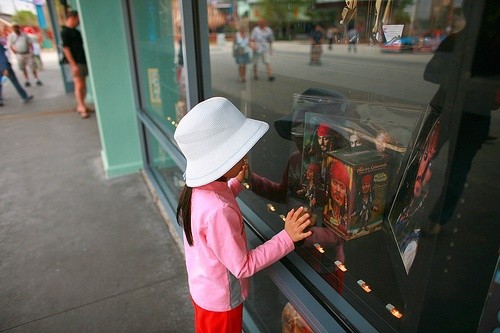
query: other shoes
79 109 89 118
22 96 34 103
24 82 31 88
36 81 42 86
268 76 274 81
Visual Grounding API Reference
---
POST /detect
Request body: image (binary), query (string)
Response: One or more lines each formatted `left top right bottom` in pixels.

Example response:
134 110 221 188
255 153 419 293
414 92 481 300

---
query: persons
355 175 378 233
61 10 89 117
248 87 353 293
309 23 324 66
311 120 340 156
299 162 321 207
24 27 43 72
174 96 313 333
233 18 278 82
395 116 439 269
11 24 44 87
323 162 349 233
348 131 364 148
373 130 391 153
0 42 35 107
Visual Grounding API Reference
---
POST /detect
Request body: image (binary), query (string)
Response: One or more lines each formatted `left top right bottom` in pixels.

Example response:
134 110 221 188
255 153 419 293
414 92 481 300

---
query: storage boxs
322 145 393 240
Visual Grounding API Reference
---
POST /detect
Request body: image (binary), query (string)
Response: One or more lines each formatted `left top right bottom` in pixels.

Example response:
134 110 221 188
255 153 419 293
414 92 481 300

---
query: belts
16 51 29 55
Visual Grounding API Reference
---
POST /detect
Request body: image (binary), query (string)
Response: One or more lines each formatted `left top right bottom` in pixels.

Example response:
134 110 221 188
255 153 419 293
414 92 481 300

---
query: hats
275 87 353 143
174 96 270 188
